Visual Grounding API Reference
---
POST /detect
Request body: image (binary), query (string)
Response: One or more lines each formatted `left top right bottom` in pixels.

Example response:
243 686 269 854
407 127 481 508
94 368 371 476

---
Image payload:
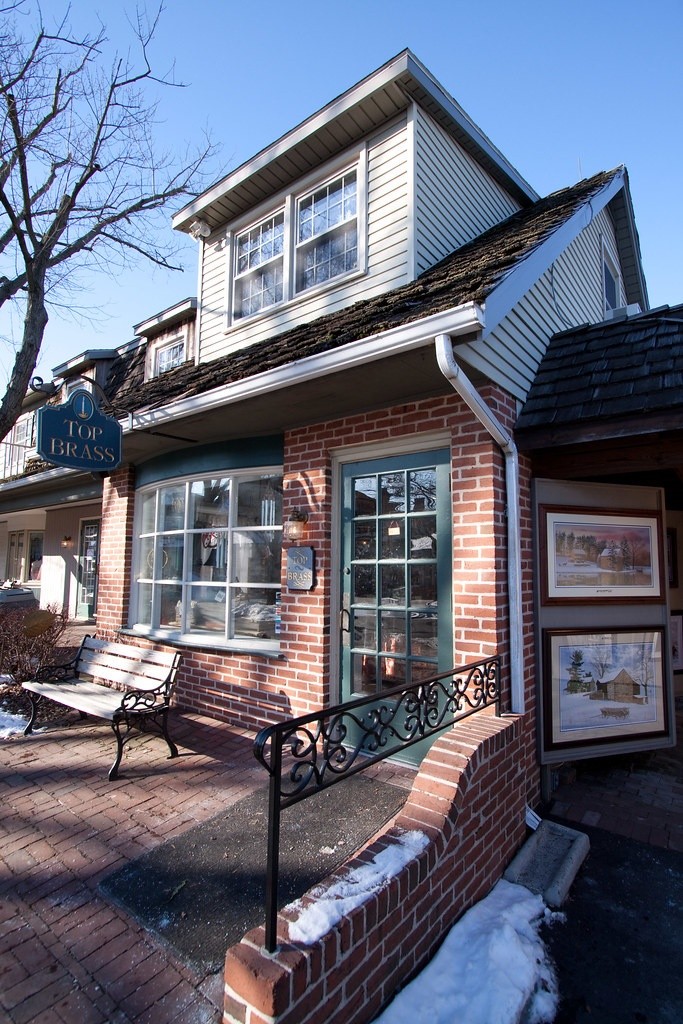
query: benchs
19 633 183 780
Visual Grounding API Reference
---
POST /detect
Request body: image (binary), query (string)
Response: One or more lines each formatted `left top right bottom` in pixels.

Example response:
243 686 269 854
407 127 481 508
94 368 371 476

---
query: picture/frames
541 622 668 752
670 609 683 675
668 527 679 588
538 501 668 605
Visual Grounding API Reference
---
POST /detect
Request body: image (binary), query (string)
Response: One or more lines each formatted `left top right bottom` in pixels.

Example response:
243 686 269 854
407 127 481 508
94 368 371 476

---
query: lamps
58 534 75 551
285 508 309 539
